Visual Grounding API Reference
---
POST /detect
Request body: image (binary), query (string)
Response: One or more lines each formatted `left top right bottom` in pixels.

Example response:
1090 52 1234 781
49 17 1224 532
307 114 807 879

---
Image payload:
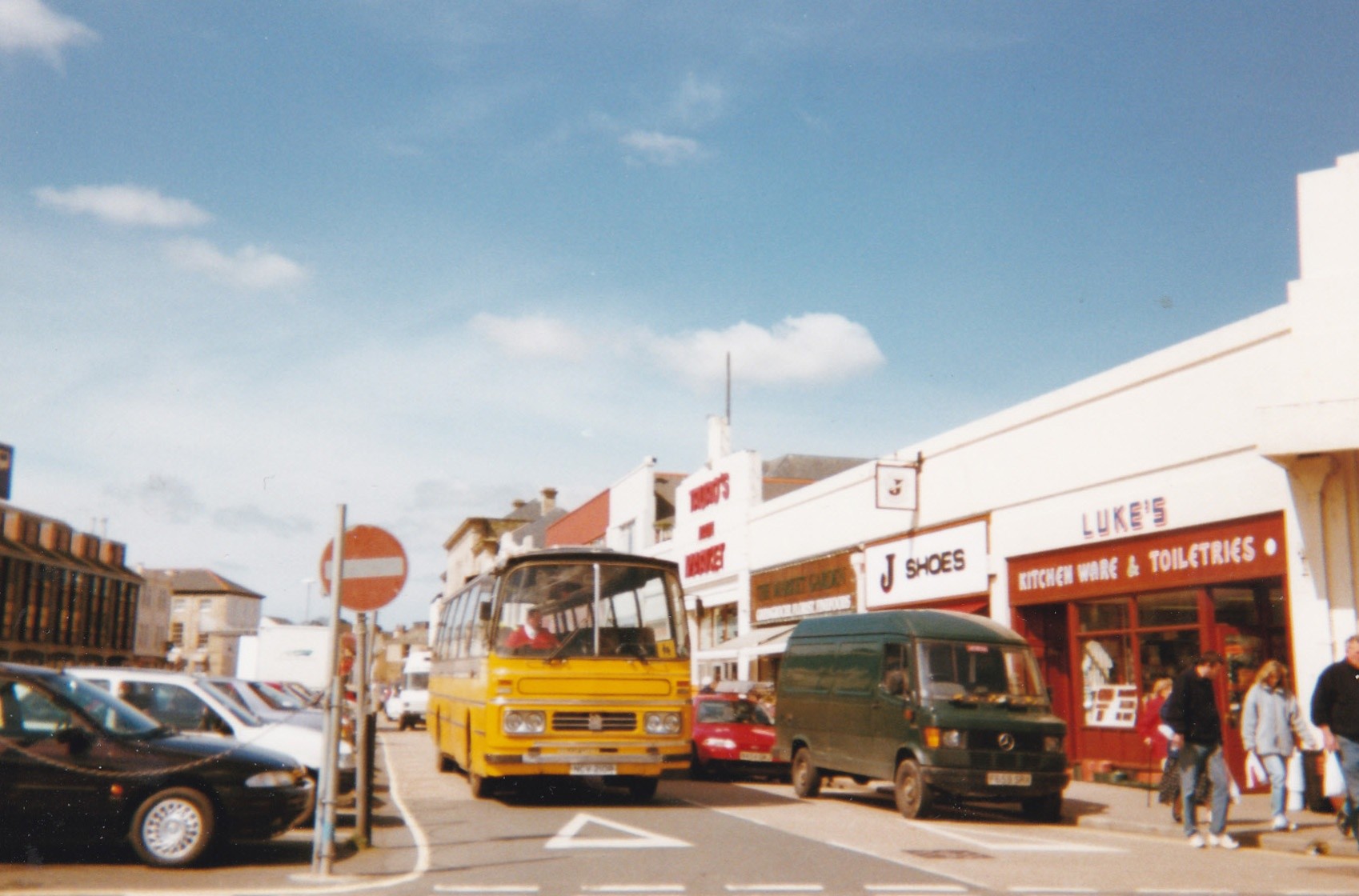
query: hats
1153 678 1174 697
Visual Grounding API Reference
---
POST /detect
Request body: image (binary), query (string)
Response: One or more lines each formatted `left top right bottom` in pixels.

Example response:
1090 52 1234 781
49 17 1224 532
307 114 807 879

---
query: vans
399 650 431 731
775 609 1071 822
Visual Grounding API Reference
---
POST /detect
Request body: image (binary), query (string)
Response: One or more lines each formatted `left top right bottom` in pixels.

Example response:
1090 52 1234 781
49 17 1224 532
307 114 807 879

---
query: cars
382 685 401 721
688 691 794 785
0 668 357 831
0 661 316 872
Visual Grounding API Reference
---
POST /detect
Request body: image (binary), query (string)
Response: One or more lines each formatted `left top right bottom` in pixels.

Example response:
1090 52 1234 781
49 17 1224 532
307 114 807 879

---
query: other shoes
1172 806 1182 823
1209 832 1240 849
1189 832 1204 847
1271 813 1287 829
1337 810 1350 836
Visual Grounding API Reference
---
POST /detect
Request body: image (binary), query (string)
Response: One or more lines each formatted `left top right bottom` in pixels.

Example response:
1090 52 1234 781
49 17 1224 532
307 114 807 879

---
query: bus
428 549 703 803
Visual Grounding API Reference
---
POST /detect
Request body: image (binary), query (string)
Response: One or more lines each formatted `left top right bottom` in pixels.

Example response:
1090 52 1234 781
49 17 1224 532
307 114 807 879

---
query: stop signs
322 524 412 612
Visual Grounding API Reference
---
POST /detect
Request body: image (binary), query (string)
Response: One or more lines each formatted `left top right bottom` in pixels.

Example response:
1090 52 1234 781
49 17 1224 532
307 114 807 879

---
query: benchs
550 625 658 657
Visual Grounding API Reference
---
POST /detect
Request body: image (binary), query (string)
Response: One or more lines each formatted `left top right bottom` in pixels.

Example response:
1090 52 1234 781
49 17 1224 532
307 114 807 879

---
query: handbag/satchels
1322 748 1347 798
1222 759 1240 805
1245 752 1268 789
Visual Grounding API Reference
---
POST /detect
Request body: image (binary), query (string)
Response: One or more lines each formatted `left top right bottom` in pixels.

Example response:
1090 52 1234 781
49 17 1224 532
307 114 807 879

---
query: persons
504 607 568 654
1159 651 1240 850
1310 634 1359 845
1237 659 1318 830
